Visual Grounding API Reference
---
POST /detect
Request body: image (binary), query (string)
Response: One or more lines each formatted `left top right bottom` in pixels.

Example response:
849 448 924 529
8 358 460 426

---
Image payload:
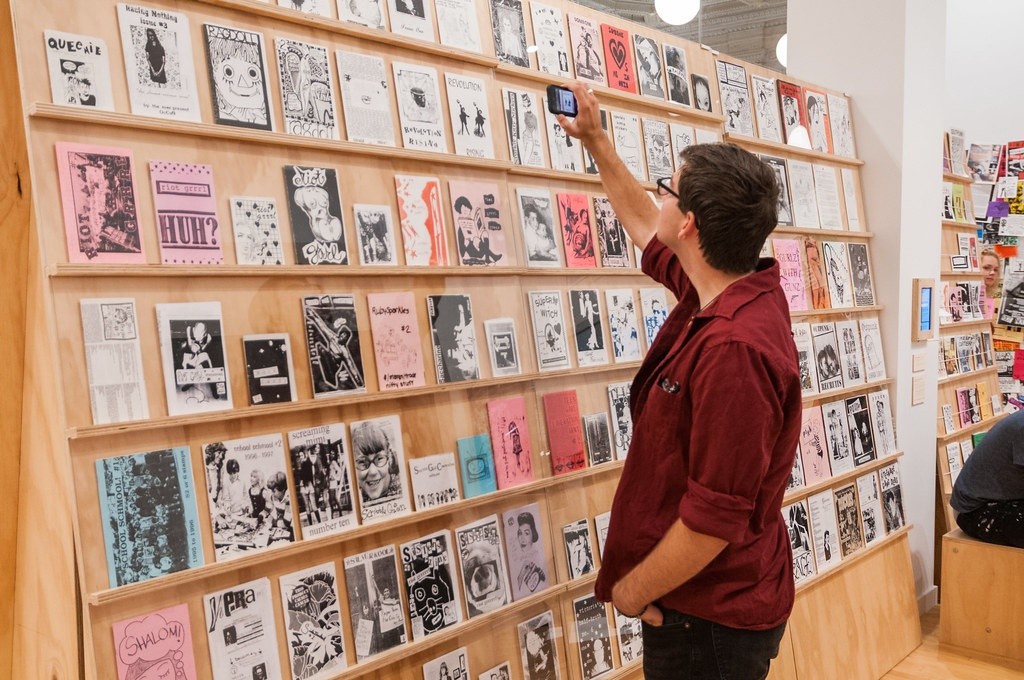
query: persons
554 75 802 680
949 409 1024 547
144 27 169 87
361 578 402 654
983 251 999 288
203 423 403 548
514 512 546 595
524 203 556 260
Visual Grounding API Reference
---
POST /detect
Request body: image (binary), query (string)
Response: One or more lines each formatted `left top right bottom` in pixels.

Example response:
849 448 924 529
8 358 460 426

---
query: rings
587 88 592 94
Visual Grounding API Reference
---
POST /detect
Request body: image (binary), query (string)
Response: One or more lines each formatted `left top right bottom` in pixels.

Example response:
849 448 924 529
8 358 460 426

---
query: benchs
938 528 1024 674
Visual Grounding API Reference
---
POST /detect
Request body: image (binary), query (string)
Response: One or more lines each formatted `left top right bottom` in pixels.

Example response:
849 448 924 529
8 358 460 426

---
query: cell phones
547 84 578 117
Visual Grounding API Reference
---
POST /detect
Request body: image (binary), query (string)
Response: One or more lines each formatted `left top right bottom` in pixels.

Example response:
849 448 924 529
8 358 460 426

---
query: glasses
657 177 700 231
356 452 389 471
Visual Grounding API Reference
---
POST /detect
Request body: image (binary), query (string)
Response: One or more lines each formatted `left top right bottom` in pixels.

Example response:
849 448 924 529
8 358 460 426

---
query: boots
326 508 331 521
314 510 321 523
307 513 312 525
